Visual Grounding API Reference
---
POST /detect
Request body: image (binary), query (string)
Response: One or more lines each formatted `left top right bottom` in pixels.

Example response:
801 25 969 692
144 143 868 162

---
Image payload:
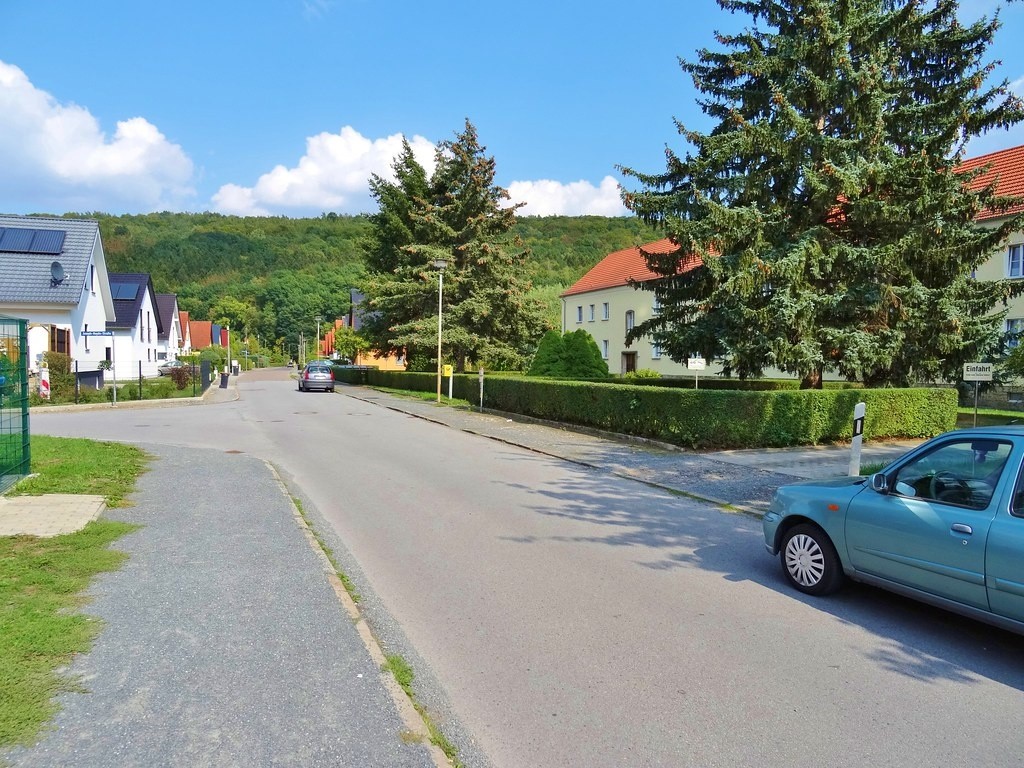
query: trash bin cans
220 372 229 388
233 366 239 376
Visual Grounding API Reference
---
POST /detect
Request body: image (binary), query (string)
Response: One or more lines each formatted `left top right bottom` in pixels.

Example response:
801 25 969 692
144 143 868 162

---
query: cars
760 424 1024 639
286 362 295 368
298 363 336 393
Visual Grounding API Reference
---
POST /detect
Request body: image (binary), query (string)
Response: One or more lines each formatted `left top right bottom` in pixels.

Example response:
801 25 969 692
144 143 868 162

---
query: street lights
314 315 322 362
433 258 448 403
